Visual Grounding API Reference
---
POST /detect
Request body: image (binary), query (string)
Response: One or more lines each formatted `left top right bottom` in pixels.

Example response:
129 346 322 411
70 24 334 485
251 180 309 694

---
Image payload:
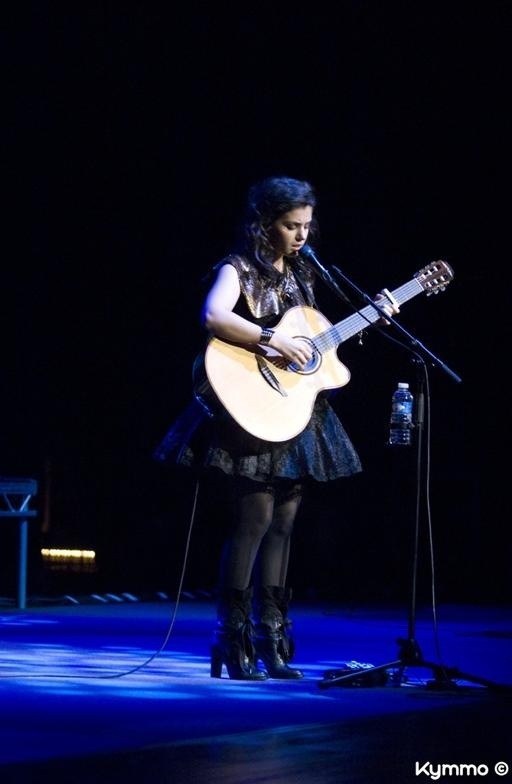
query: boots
210 588 268 680
254 585 304 679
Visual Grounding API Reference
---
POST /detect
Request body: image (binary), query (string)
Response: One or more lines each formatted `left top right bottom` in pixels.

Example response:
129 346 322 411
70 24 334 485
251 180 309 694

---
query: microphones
298 244 338 290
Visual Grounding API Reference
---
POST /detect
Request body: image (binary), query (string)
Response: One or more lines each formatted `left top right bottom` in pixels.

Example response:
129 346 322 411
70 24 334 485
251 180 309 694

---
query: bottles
390 382 415 444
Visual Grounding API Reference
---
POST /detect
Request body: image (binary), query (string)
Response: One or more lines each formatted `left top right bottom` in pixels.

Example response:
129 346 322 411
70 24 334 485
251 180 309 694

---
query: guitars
191 259 454 453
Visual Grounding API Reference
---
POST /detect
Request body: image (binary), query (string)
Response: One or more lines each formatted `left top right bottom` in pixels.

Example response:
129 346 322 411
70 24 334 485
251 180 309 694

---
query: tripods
320 262 501 689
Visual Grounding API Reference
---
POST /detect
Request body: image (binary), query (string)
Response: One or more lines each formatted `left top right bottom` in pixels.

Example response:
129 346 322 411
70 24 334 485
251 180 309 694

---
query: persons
141 174 404 683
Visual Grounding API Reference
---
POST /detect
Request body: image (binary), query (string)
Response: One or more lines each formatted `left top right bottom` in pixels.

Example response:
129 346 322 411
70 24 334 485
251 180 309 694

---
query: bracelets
258 328 276 346
